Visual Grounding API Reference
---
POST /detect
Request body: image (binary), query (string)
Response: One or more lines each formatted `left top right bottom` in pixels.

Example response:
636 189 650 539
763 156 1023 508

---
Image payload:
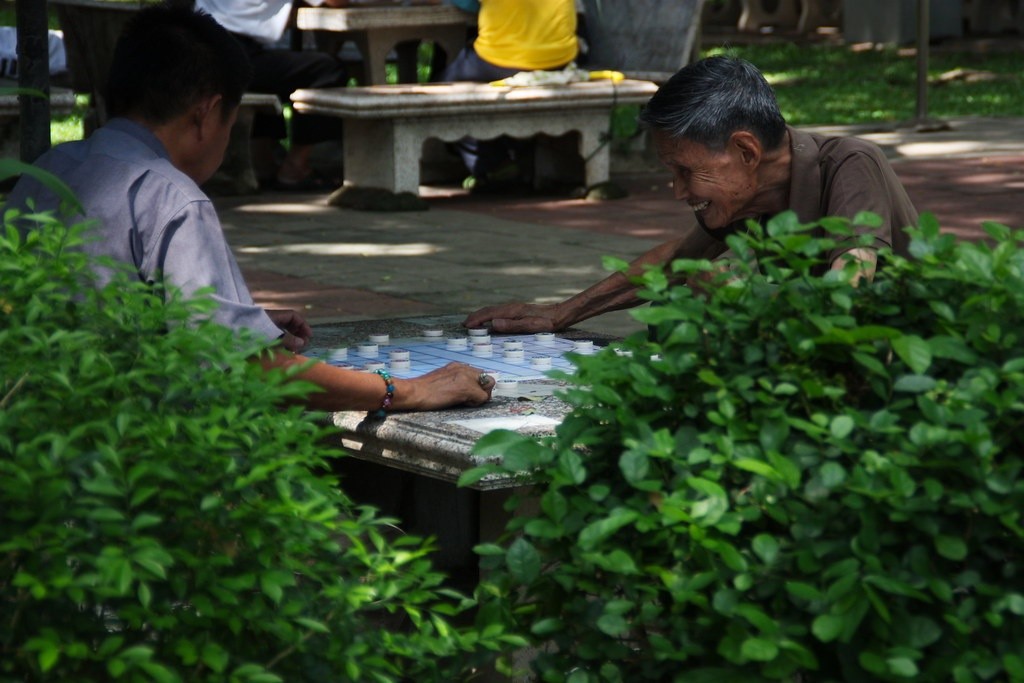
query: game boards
299 332 664 381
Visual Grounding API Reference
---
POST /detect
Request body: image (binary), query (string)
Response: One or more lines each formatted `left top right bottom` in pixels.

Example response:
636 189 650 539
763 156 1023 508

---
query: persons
462 52 963 407
1 0 496 417
445 0 578 184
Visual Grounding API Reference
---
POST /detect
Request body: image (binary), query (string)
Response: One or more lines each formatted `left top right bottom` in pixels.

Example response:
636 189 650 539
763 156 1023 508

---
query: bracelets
372 368 395 417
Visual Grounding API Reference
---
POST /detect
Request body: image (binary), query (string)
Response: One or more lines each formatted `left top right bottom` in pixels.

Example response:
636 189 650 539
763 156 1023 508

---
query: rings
478 371 489 386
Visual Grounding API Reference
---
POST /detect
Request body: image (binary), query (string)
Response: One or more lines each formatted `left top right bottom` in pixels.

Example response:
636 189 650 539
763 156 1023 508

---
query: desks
298 3 476 86
301 313 625 545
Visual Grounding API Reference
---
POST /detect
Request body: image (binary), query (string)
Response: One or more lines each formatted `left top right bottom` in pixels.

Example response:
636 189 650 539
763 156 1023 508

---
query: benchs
290 73 658 212
86 89 281 191
